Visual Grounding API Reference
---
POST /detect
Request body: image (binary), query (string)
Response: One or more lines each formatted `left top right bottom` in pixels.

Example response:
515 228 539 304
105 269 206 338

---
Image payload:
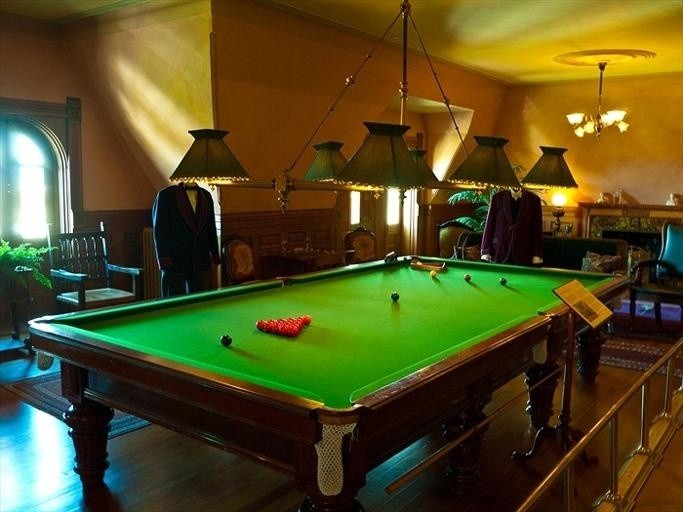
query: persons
151 179 221 298
481 185 544 267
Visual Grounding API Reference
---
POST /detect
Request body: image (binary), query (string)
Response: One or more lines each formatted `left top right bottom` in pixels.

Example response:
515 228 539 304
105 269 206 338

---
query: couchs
448 228 628 291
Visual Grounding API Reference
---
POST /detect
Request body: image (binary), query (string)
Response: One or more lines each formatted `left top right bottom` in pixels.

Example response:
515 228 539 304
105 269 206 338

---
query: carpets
5 371 154 440
567 331 681 382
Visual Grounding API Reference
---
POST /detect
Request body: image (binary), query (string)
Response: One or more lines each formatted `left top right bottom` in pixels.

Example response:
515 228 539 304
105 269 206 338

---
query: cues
385 359 579 494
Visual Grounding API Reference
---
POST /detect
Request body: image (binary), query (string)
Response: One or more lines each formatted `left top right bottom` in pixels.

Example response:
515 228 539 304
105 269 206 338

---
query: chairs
343 223 376 265
219 236 260 286
631 217 681 332
434 220 474 260
46 227 143 314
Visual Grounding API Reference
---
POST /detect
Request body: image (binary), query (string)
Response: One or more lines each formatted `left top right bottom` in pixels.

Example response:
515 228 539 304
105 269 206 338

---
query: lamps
160 0 575 213
566 59 629 136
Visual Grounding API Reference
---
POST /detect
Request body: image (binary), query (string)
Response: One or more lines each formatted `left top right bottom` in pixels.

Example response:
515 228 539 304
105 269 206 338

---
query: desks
256 246 355 274
30 254 626 512
0 295 37 357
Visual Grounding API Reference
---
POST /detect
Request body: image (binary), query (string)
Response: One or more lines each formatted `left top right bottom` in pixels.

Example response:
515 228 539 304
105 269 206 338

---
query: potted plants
0 238 59 299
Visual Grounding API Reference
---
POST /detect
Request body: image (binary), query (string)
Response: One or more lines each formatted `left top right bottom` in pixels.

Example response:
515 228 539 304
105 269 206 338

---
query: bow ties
182 184 199 192
511 191 524 200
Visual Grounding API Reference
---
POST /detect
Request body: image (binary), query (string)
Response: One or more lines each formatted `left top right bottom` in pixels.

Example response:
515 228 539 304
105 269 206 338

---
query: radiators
138 225 161 299
